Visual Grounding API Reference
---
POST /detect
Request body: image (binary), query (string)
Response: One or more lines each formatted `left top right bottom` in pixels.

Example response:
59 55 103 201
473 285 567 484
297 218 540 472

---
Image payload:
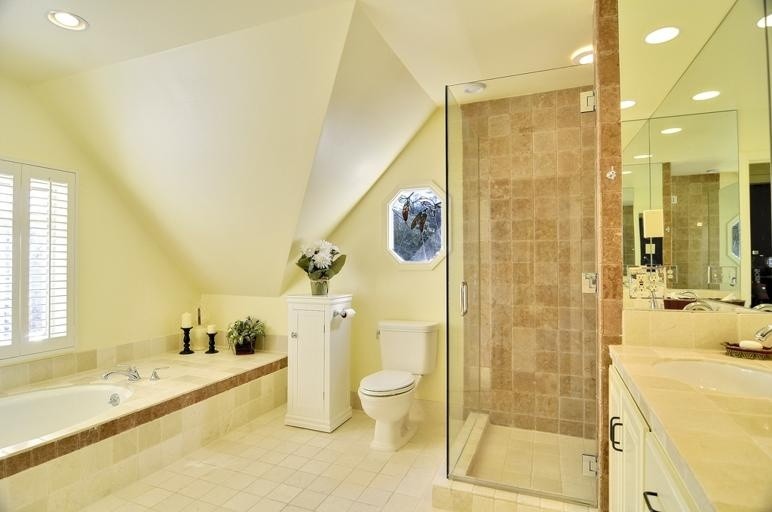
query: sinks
649 356 772 410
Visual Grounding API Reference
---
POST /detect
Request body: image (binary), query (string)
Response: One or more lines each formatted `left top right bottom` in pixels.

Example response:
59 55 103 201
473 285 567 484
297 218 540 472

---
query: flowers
225 316 266 355
295 238 346 287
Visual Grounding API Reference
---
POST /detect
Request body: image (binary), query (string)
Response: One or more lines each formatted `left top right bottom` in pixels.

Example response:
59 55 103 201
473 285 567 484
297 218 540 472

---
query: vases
235 336 256 355
310 278 328 295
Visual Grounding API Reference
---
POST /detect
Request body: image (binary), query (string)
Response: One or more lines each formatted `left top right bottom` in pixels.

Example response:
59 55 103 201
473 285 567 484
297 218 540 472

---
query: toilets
358 321 439 453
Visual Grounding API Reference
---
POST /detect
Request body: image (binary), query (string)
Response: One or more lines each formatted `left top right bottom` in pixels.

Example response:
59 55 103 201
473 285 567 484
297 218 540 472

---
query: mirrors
620 109 750 311
651 0 770 314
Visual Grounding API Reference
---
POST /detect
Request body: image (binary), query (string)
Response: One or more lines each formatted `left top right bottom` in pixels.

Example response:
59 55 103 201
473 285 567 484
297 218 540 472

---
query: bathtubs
0 368 204 454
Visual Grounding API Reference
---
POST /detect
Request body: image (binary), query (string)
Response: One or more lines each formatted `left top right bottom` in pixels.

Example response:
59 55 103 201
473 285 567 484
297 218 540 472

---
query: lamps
640 208 665 272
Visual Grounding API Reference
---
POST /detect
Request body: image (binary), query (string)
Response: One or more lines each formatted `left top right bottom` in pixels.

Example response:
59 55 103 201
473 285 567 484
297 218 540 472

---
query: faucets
754 323 772 342
101 366 141 381
753 303 772 312
682 301 715 311
679 291 701 300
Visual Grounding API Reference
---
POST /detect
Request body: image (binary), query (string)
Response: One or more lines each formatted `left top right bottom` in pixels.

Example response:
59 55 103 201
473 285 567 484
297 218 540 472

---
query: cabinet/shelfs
284 291 355 433
636 430 701 512
595 361 655 511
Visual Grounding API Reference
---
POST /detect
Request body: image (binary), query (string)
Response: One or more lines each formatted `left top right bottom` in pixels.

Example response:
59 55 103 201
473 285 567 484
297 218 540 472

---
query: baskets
662 296 698 309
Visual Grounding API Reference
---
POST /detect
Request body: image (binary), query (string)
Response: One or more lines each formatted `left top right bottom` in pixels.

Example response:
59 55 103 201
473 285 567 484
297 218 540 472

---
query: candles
207 324 216 334
179 311 193 328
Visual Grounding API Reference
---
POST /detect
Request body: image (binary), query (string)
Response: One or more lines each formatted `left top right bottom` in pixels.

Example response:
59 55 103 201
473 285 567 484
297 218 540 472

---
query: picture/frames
382 177 453 273
